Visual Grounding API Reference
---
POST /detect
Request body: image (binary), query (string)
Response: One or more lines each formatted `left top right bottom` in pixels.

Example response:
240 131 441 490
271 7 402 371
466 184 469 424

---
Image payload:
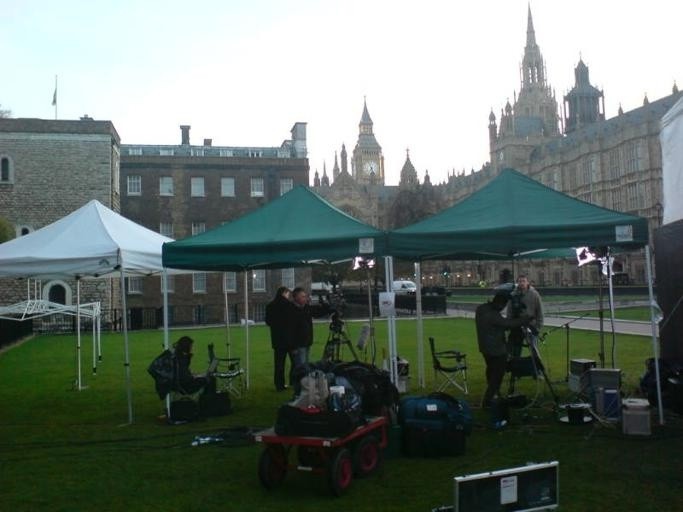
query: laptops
193 358 219 378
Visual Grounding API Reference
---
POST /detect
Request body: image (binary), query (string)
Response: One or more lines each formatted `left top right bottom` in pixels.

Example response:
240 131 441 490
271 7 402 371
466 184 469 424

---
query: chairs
429 337 469 394
207 342 245 397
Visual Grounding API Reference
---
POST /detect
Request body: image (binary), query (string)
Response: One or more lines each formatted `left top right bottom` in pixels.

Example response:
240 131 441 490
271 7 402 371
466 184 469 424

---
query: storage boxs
430 459 559 512
568 357 651 438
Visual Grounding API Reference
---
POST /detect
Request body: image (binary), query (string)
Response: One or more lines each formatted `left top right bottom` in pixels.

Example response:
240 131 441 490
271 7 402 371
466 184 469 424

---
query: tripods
321 315 359 362
505 317 559 402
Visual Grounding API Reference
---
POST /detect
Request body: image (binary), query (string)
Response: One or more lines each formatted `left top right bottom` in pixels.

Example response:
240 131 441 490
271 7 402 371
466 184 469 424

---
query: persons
475 290 525 409
506 275 544 357
265 286 313 392
173 336 216 396
494 268 515 293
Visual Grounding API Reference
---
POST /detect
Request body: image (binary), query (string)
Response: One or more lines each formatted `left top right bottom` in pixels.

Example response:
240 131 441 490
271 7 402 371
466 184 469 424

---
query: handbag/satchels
398 391 473 457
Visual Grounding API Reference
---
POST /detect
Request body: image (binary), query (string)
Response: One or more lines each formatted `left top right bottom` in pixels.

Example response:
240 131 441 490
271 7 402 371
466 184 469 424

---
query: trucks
310 282 332 305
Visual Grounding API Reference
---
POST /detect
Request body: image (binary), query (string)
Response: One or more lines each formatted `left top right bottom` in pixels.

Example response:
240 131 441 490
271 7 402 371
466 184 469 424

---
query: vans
393 281 416 295
